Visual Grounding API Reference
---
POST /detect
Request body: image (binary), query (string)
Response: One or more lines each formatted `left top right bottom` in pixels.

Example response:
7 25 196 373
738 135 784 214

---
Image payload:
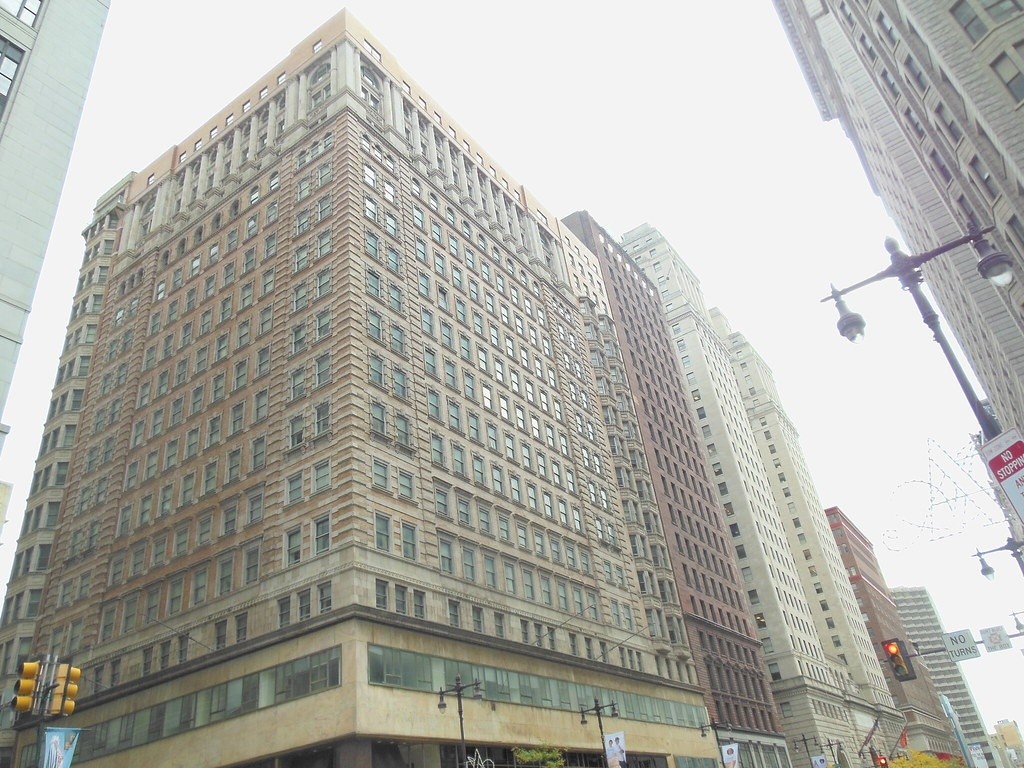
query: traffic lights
878 757 889 768
50 663 81 717
10 660 45 729
880 637 918 683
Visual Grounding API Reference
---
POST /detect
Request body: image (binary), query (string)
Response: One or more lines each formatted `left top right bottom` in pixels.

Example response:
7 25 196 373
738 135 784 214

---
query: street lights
436 672 482 767
972 537 1024 581
700 717 732 768
793 733 819 768
819 739 844 768
579 697 619 768
859 748 882 768
1009 609 1024 634
816 222 1024 533
